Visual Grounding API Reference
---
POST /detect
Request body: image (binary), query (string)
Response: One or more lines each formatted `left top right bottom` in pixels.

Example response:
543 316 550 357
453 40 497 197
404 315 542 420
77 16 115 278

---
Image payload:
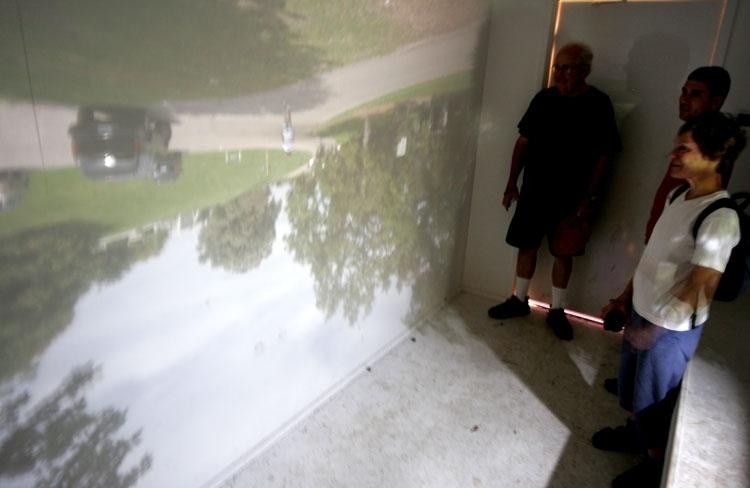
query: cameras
604 308 625 332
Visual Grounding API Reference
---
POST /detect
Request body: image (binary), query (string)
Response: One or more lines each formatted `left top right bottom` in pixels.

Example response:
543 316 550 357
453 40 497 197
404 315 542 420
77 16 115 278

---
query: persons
488 40 623 339
588 111 741 488
278 102 296 159
600 65 734 399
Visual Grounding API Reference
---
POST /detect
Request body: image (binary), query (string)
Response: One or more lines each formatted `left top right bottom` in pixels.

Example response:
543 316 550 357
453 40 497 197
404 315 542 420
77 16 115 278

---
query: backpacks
670 184 747 304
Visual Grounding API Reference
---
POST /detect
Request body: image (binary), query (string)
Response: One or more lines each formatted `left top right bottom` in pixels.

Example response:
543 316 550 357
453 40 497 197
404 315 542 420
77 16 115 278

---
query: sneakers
610 456 663 487
487 294 531 320
593 418 644 456
545 307 574 341
604 377 619 396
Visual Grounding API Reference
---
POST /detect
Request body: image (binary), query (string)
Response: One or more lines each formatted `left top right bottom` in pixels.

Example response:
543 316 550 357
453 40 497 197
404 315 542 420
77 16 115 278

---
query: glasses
550 62 576 77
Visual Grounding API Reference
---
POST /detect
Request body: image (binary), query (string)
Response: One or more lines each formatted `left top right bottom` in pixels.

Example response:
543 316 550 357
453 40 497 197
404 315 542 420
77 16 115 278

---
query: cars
65 103 171 178
0 168 32 211
142 150 182 184
